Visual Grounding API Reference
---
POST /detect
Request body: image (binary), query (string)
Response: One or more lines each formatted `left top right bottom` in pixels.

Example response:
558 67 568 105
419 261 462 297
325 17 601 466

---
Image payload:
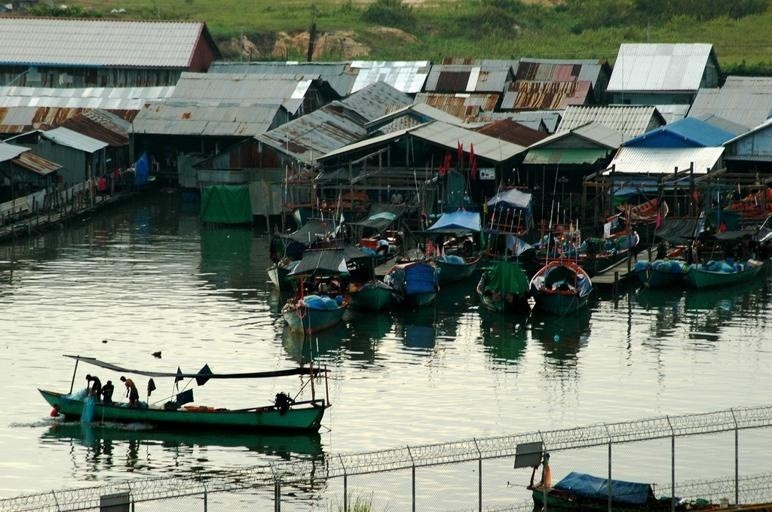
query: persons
85 373 101 397
120 375 140 410
113 165 123 184
541 451 553 486
101 380 115 405
96 175 107 204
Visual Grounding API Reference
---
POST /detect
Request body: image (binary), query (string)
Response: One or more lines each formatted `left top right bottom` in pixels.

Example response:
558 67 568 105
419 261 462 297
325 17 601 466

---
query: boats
264 177 640 317
618 196 669 232
279 272 349 335
532 472 681 511
34 354 331 431
633 176 771 290
